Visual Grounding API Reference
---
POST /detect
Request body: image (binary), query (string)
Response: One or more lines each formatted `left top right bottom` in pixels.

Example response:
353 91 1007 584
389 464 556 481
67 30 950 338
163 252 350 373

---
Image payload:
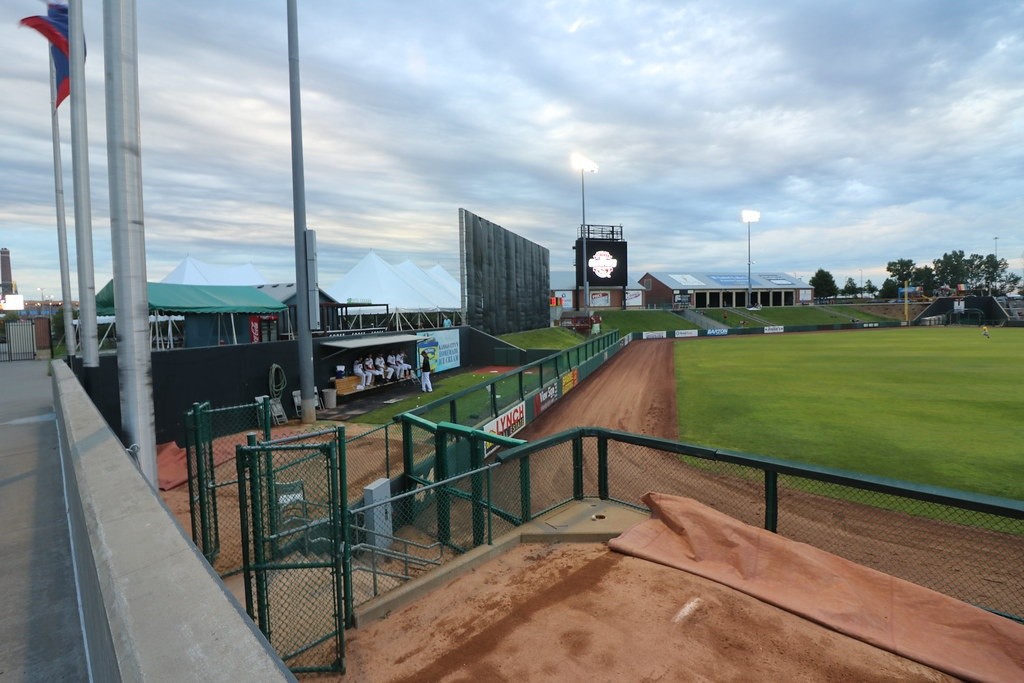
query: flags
20 0 87 108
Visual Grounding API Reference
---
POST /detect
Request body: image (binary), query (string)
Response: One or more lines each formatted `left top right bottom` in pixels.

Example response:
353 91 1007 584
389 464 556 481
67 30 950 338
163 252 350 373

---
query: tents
55 252 467 351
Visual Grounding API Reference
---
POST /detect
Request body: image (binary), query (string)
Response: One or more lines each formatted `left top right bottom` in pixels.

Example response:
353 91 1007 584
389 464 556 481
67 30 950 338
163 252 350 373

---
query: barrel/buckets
322 388 336 409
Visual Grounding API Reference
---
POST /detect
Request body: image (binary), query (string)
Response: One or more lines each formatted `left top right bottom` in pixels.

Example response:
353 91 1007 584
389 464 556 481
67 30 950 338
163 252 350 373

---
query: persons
354 349 412 388
983 324 990 338
443 316 451 328
724 311 728 320
416 351 432 393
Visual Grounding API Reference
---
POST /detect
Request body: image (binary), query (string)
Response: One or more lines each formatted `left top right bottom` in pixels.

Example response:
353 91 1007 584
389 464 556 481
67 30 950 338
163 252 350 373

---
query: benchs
335 367 415 397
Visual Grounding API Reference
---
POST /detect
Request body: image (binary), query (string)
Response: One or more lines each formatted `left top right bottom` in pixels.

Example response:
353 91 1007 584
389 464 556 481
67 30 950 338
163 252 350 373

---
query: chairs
269 386 325 426
276 478 309 529
